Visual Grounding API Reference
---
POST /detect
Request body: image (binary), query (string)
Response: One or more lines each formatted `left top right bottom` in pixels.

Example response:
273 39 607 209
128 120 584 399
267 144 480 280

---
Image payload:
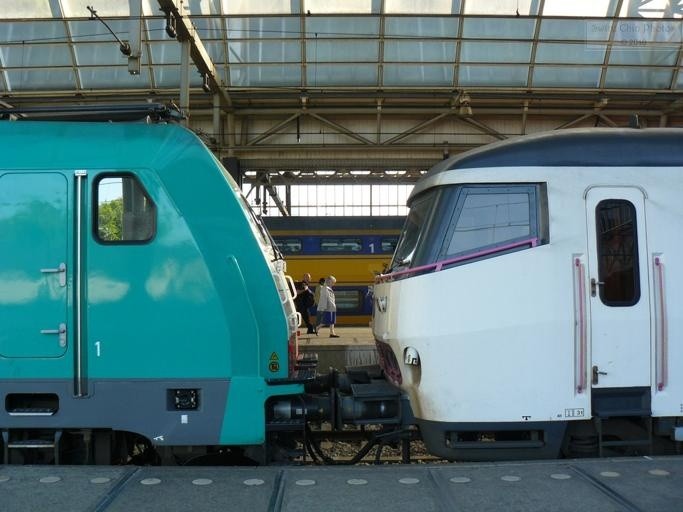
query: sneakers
307 324 340 337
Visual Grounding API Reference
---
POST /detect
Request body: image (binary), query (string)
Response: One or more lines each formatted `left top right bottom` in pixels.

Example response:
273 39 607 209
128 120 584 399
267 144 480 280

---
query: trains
258 216 410 326
0 101 683 465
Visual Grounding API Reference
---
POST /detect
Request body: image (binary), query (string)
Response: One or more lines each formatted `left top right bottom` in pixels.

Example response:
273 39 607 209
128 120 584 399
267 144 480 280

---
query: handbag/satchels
304 289 314 307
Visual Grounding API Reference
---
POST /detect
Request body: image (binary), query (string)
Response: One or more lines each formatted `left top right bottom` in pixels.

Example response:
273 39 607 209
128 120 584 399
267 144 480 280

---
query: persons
313 278 325 330
314 275 339 337
292 272 315 334
